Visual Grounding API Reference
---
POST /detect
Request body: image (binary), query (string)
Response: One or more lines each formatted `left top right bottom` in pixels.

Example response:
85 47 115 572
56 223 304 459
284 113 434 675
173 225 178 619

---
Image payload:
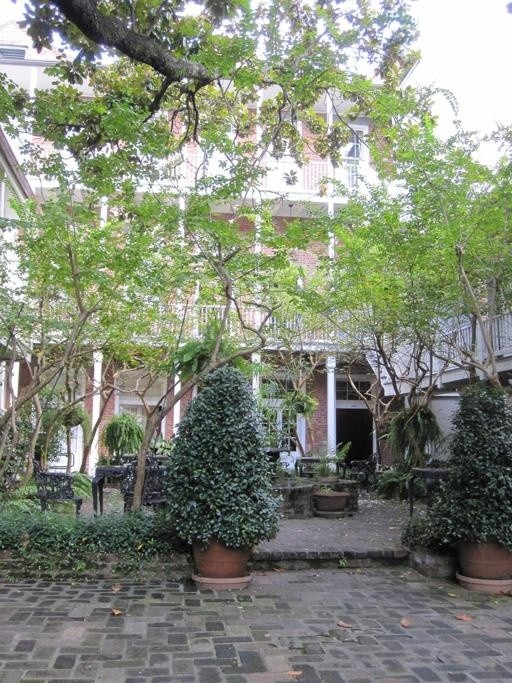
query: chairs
33 460 83 520
121 454 167 513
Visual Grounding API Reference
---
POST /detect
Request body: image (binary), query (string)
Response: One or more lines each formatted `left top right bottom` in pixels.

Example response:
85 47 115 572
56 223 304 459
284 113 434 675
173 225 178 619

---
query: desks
408 467 451 518
92 466 129 517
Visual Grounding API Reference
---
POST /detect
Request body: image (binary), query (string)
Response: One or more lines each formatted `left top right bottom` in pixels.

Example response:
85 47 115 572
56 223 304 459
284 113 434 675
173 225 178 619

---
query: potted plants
164 367 285 578
425 382 512 579
311 486 349 512
